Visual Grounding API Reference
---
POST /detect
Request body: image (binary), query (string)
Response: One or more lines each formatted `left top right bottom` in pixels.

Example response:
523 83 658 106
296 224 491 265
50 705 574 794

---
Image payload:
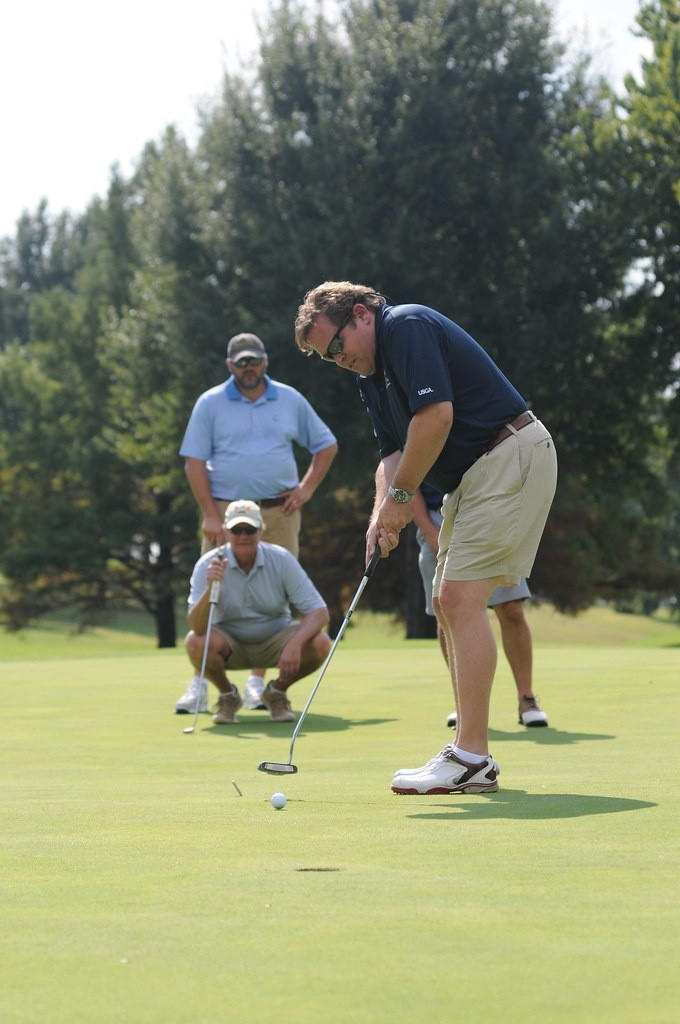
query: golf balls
271 792 288 809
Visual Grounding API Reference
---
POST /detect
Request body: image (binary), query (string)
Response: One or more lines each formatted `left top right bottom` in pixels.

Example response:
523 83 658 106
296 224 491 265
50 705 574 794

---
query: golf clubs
179 548 224 737
256 538 382 777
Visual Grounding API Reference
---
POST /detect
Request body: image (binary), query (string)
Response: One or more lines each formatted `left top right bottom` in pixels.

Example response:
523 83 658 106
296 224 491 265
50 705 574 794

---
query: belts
213 497 286 510
426 503 443 514
471 411 537 464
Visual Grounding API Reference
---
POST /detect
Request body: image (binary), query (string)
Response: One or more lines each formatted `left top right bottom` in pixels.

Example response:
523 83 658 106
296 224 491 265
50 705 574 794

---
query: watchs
388 485 415 503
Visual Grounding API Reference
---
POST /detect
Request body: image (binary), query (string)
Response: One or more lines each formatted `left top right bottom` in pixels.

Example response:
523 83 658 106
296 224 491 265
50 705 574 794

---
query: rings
401 524 406 529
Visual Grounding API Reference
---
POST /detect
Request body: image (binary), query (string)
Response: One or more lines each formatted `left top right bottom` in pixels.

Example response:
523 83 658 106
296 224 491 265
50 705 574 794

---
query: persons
175 332 338 716
184 499 332 723
291 279 557 793
411 481 548 730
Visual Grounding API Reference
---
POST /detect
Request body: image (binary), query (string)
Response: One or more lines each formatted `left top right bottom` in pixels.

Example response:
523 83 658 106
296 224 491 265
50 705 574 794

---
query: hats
226 333 265 363
224 498 262 530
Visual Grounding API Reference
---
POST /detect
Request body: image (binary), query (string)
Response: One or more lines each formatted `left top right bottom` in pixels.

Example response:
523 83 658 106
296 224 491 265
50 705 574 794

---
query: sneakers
175 680 207 713
262 680 296 722
242 677 267 709
390 744 500 795
518 694 548 727
448 710 456 727
211 684 243 724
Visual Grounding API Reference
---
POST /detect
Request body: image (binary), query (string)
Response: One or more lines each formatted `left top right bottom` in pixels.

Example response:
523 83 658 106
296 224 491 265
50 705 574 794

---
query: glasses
235 357 263 368
321 297 365 362
230 526 257 535
